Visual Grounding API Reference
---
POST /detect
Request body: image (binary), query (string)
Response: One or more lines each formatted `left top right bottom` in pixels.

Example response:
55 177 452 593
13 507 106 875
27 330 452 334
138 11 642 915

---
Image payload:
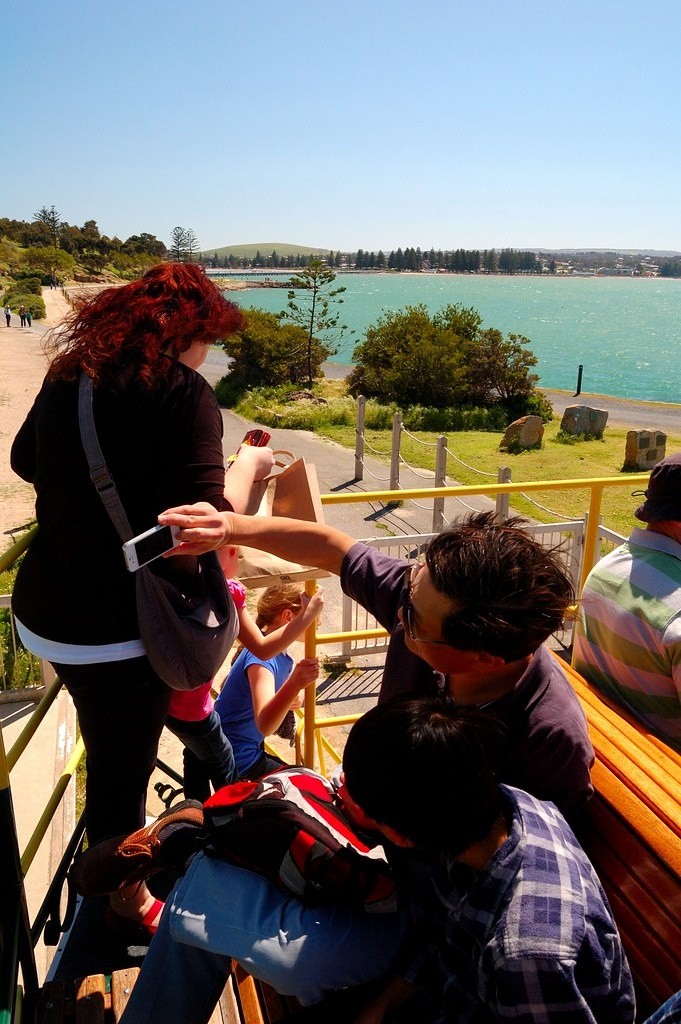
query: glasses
402 562 450 646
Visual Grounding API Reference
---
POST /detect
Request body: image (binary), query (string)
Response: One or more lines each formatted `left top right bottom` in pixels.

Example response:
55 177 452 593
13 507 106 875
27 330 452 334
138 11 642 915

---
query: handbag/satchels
136 551 240 691
238 450 332 588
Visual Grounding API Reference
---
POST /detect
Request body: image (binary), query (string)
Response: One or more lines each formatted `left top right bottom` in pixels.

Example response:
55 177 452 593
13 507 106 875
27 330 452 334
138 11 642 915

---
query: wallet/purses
226 429 271 473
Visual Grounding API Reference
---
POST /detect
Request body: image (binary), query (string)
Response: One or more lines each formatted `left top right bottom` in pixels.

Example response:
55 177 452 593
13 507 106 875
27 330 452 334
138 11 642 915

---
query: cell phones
122 522 181 574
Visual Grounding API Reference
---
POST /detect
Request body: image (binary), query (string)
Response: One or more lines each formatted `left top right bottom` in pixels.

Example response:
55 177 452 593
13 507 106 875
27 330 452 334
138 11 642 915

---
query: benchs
232 643 681 1024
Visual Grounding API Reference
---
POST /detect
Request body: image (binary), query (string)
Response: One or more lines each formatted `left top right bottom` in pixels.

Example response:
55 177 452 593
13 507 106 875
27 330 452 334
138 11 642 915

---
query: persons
4 261 275 938
572 457 681 746
65 502 681 1024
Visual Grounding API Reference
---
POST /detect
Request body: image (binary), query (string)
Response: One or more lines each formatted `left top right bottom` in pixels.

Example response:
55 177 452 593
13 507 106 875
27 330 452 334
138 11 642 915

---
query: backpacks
202 764 417 905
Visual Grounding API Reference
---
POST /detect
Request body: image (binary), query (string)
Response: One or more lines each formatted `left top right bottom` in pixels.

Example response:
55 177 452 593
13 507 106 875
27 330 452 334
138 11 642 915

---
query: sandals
105 880 166 946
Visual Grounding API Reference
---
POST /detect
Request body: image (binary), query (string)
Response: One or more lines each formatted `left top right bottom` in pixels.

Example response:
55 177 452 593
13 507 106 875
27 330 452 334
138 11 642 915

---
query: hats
631 453 681 523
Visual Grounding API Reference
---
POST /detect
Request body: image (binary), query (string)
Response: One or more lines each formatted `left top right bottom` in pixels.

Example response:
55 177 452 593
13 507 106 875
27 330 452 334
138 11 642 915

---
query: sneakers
70 799 205 896
